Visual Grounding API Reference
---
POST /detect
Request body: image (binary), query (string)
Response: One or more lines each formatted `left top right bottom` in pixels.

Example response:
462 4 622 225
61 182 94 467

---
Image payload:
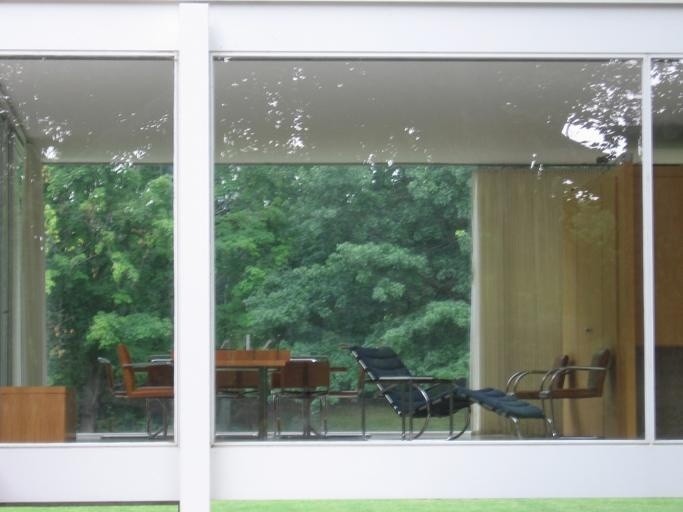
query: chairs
95 342 367 440
505 355 570 440
536 348 611 441
345 344 558 441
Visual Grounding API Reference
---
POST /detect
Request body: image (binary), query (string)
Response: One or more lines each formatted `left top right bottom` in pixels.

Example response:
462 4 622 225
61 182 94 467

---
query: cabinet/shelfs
0 384 77 443
